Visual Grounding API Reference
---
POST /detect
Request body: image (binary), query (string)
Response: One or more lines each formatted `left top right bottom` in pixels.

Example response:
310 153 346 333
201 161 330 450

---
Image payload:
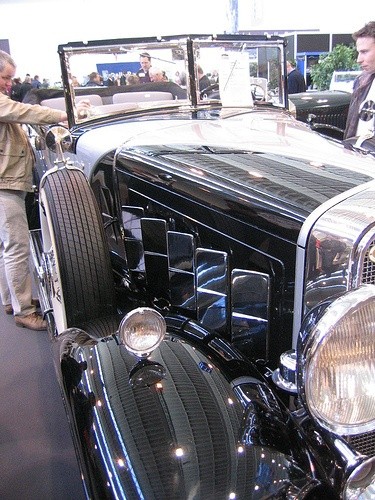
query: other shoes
15 312 47 331
5 298 40 315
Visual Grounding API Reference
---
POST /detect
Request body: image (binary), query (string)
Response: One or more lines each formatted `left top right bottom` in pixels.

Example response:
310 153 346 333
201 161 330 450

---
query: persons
0 49 91 331
309 234 338 282
194 63 212 99
71 72 128 87
9 73 50 102
287 59 306 95
343 21 375 139
135 52 168 83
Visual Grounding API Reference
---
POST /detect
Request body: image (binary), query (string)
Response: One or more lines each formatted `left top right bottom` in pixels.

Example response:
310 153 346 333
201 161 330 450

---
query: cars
17 30 375 500
